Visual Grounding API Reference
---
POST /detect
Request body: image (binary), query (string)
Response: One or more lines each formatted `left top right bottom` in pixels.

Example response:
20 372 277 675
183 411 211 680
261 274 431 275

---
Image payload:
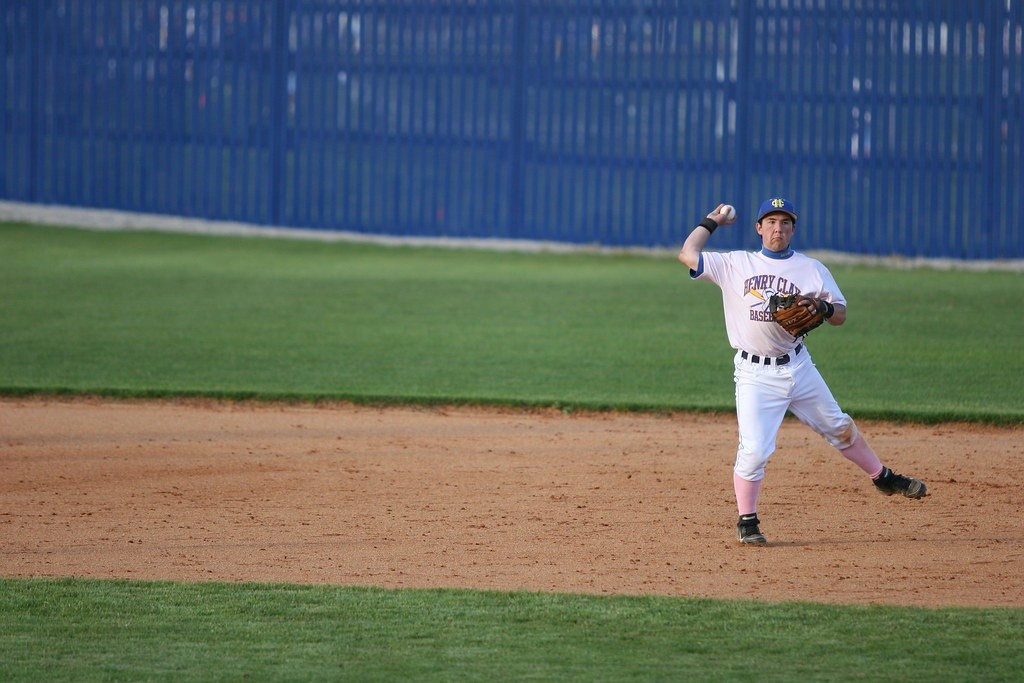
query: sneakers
872 466 925 498
737 513 766 544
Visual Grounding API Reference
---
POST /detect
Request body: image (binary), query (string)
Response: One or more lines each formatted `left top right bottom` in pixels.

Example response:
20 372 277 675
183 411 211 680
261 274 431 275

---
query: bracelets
822 301 834 319
698 217 718 236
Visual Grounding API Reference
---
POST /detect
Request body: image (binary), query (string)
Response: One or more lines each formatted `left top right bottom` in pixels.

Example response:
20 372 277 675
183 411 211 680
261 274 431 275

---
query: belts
742 344 801 365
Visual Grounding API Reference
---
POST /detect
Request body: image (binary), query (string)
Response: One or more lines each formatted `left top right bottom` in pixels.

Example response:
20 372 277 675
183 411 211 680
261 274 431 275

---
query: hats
756 196 798 223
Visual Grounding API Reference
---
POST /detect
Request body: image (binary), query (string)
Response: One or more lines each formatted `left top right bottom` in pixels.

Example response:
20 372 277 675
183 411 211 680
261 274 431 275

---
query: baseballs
720 204 735 221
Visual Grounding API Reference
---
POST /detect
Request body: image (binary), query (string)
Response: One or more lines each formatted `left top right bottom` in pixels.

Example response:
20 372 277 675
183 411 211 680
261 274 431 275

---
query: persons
679 197 926 545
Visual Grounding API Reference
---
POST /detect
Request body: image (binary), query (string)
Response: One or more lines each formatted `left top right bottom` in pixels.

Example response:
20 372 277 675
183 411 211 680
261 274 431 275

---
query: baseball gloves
769 292 834 338
